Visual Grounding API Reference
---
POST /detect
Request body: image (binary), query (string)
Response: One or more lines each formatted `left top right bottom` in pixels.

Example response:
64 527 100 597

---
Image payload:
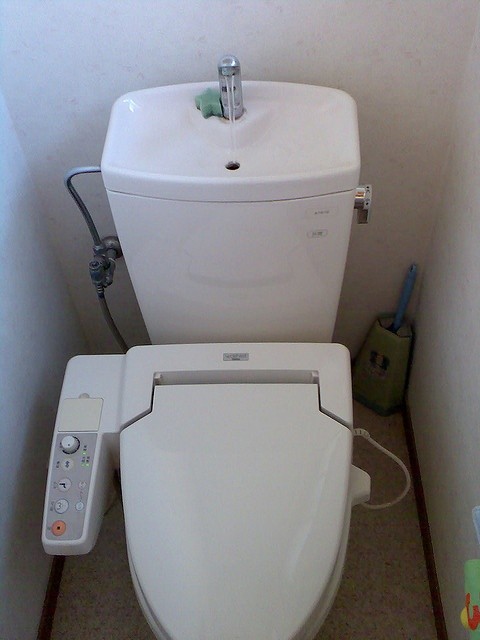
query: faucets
217 54 245 122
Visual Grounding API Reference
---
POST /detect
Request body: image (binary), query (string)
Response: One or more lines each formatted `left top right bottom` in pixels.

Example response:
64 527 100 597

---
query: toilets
39 79 372 640
